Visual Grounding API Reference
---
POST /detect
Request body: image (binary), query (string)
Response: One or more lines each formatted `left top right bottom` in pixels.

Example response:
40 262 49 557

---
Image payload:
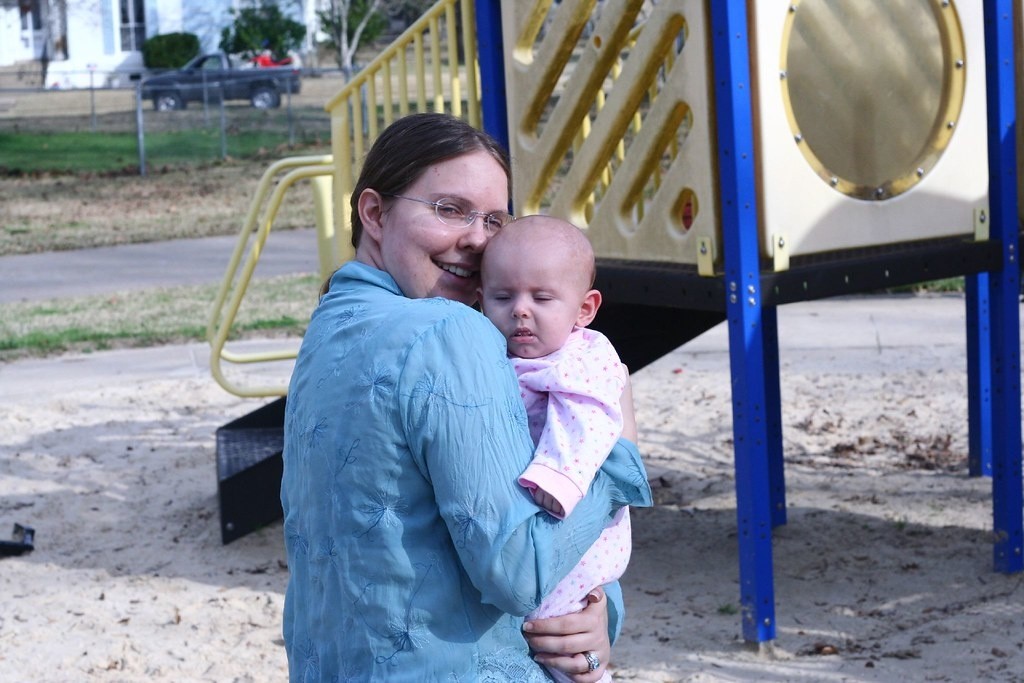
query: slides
203 0 1024 551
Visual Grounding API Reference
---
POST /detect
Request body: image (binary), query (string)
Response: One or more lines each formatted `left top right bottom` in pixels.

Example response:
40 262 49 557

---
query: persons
474 215 632 683
280 112 639 683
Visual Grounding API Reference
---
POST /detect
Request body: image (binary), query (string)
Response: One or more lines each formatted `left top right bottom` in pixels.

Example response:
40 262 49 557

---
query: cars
232 53 303 78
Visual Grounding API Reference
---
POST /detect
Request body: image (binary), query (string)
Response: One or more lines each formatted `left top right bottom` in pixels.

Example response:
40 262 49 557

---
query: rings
583 650 601 672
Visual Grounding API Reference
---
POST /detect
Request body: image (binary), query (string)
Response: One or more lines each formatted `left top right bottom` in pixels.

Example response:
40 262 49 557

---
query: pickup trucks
137 50 300 114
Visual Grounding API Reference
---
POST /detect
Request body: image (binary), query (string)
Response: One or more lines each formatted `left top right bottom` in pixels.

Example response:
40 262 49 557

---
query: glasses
378 188 516 237
